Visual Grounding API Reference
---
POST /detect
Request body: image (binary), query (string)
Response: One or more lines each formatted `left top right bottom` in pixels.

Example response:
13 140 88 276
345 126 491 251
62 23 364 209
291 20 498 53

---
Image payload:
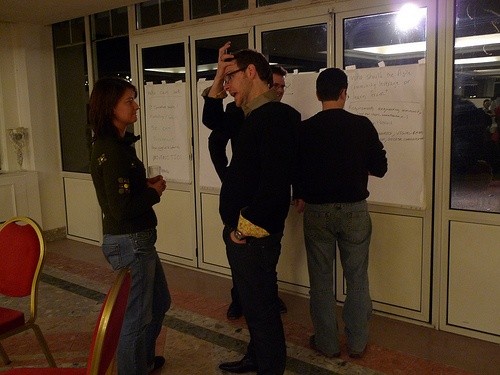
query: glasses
224 63 258 84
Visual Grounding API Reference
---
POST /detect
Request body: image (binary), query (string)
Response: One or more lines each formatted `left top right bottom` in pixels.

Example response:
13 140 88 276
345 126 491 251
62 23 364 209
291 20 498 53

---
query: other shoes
278 297 287 313
308 334 341 358
146 356 164 371
219 354 255 374
349 344 367 358
226 305 241 317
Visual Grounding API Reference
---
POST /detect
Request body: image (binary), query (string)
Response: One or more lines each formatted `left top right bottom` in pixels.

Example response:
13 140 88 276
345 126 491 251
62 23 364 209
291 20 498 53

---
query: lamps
7 127 26 169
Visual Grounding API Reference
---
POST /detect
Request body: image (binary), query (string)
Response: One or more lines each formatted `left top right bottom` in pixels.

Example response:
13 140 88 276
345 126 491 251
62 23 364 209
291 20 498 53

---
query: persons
88 77 172 375
482 97 500 127
209 66 301 319
293 68 389 358
201 42 295 375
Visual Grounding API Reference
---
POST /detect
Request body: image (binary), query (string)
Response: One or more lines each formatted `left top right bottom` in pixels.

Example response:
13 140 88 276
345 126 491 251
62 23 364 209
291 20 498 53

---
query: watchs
234 229 246 240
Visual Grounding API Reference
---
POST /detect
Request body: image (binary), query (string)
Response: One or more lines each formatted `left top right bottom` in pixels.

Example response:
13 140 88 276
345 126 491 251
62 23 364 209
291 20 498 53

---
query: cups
147 166 162 184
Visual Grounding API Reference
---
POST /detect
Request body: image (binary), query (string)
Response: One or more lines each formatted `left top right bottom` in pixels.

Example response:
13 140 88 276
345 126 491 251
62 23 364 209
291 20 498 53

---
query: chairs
0 217 56 367
0 268 132 375
